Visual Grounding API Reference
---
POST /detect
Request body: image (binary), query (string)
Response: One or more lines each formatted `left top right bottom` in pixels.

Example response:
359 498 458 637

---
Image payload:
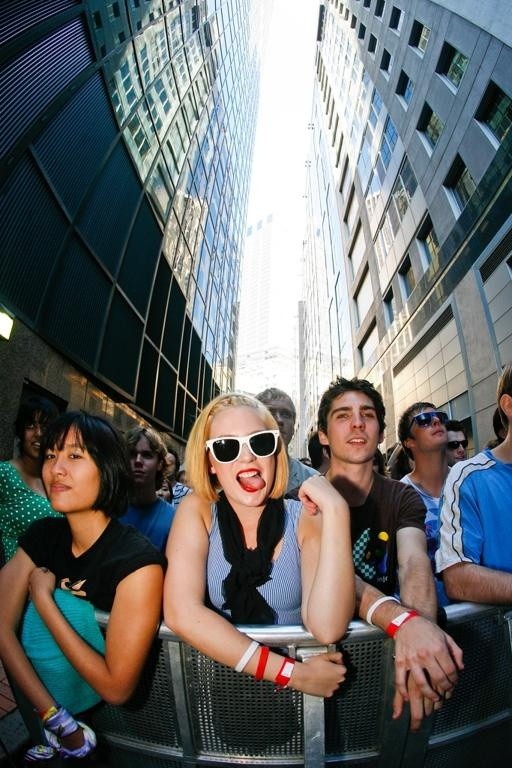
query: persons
0 362 512 768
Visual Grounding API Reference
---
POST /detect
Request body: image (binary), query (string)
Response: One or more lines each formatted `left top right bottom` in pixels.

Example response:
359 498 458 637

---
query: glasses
447 440 468 449
408 411 450 431
205 429 280 464
